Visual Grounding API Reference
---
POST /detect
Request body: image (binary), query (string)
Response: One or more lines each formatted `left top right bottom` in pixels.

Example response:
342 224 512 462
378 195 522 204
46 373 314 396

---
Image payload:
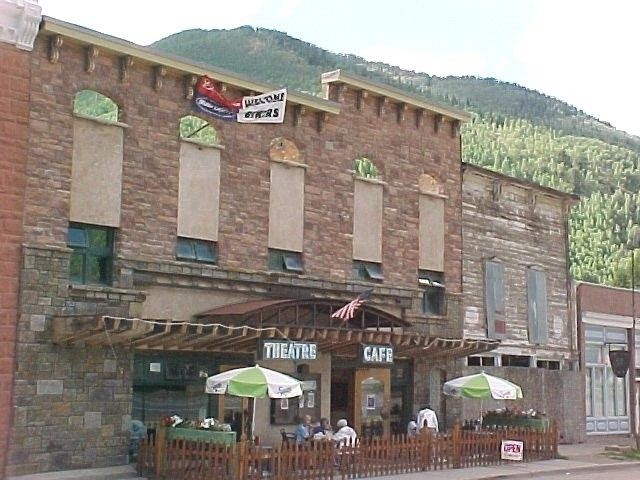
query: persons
295 414 316 445
313 417 335 434
323 418 360 466
406 415 418 437
416 403 440 434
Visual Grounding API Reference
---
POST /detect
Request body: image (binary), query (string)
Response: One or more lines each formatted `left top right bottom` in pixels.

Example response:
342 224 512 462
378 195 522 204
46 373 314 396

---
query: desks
460 430 495 456
436 434 452 440
483 416 553 431
250 446 272 471
307 436 340 462
147 426 237 475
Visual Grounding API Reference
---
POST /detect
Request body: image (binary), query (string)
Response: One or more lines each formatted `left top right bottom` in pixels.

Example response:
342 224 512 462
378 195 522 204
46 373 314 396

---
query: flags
332 288 372 322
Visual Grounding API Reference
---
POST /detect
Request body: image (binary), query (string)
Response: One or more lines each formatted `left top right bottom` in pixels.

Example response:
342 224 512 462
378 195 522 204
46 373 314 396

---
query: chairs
281 428 297 472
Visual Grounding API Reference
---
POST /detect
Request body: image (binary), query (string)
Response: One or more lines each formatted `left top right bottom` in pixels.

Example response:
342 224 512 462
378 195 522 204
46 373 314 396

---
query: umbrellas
442 369 526 434
202 364 301 443
